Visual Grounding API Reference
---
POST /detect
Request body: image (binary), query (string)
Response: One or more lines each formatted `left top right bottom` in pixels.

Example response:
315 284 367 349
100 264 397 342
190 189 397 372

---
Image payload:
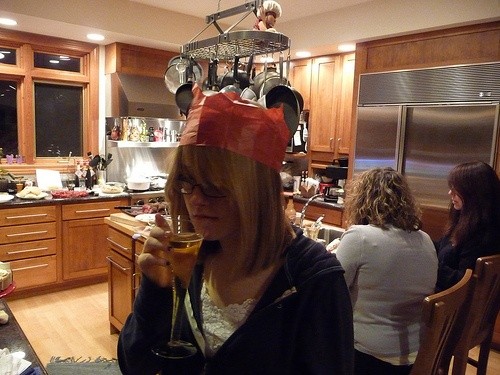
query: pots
165 53 304 138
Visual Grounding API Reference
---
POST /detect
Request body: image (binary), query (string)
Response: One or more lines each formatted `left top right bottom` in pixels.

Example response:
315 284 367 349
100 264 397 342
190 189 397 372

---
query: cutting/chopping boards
35 169 63 191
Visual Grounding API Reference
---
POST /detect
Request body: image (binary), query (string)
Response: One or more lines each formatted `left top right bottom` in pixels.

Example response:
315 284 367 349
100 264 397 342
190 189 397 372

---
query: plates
135 213 157 223
15 191 48 200
0 191 14 203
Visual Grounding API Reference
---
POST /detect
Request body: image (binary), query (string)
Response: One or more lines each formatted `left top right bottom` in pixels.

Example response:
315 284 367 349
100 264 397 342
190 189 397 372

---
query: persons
331 165 438 375
253 0 282 32
433 160 500 310
117 83 355 375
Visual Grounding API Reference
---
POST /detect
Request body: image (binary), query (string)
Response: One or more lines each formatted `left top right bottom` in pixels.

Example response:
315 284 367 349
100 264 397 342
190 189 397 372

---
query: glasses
172 176 234 198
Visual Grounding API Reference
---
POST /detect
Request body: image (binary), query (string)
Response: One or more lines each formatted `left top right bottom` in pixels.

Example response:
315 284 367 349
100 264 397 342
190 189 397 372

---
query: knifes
113 205 143 209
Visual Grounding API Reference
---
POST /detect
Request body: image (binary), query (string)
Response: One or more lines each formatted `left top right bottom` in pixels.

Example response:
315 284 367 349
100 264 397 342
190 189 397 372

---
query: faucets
299 193 325 230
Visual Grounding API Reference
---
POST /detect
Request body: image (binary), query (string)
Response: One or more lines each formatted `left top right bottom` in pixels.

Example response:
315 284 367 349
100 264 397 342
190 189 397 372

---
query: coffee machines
320 164 348 202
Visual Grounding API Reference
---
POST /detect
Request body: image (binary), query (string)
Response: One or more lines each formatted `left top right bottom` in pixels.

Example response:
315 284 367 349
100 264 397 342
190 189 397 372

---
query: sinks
301 227 345 245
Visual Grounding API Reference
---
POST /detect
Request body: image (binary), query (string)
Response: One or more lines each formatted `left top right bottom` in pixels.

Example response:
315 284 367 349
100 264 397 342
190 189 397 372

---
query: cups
300 222 321 242
60 171 77 190
96 170 107 185
332 157 349 167
293 175 301 193
16 155 25 164
6 154 14 165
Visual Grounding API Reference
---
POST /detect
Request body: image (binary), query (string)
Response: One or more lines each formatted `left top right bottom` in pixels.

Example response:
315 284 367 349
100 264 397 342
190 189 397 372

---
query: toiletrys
284 199 296 226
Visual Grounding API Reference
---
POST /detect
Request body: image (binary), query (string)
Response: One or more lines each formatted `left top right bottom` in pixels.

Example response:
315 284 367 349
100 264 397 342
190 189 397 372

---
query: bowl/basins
102 182 125 194
127 178 151 191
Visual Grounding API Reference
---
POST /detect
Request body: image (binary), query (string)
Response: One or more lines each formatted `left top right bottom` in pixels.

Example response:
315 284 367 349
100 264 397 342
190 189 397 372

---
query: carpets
47 356 123 375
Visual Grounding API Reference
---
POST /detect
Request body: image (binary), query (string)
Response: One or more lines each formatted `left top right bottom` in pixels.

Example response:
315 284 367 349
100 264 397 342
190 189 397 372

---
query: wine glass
152 214 209 358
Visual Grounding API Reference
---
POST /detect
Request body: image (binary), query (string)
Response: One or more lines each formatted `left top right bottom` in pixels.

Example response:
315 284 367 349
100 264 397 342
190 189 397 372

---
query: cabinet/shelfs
0 52 356 335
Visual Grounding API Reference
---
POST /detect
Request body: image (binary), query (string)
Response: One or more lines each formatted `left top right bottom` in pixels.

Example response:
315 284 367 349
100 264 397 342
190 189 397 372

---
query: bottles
76 157 94 195
285 195 297 227
109 125 181 143
278 160 309 193
7 177 33 194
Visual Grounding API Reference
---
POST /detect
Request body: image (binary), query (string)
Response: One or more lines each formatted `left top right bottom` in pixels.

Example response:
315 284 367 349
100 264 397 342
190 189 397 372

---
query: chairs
453 255 500 375
410 269 473 375
